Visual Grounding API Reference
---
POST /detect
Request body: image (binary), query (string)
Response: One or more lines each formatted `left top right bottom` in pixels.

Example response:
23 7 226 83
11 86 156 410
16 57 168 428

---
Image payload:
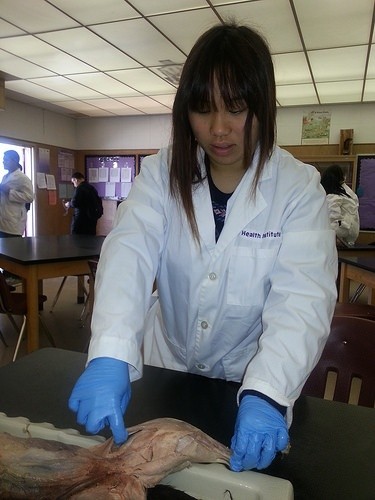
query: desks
0 234 375 500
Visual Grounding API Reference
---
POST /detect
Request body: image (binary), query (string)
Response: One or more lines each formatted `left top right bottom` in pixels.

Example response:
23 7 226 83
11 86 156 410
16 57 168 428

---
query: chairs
301 316 375 409
0 271 56 362
80 260 98 329
334 303 375 321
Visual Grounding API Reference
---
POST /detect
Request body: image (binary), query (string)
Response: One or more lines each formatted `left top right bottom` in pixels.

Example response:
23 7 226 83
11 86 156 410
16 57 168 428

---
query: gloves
229 395 289 472
68 358 131 445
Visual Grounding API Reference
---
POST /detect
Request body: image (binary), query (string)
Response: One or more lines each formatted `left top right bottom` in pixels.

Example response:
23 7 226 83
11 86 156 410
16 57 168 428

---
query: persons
320 166 360 249
0 150 35 291
65 171 104 237
67 20 339 472
